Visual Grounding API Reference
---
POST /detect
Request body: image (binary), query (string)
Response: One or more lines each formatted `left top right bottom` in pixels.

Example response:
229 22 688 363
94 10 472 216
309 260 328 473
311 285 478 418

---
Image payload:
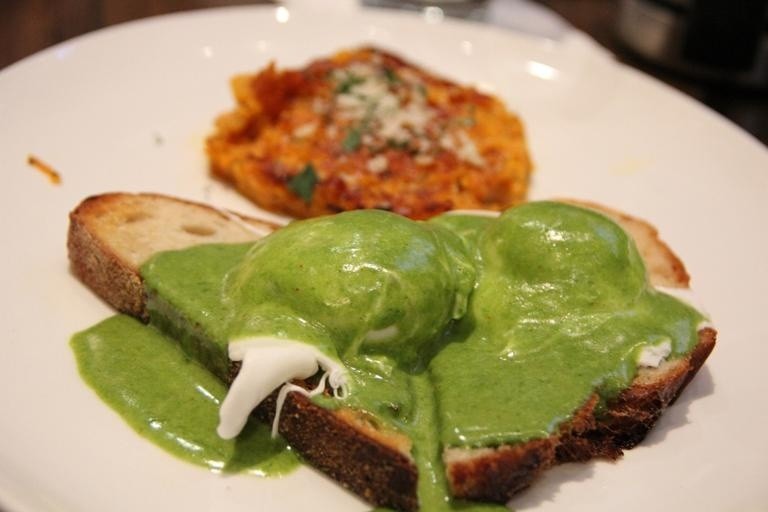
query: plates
0 1 766 512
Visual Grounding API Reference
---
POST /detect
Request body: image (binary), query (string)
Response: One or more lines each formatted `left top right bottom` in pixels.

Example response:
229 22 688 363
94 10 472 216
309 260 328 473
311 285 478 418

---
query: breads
68 192 715 512
